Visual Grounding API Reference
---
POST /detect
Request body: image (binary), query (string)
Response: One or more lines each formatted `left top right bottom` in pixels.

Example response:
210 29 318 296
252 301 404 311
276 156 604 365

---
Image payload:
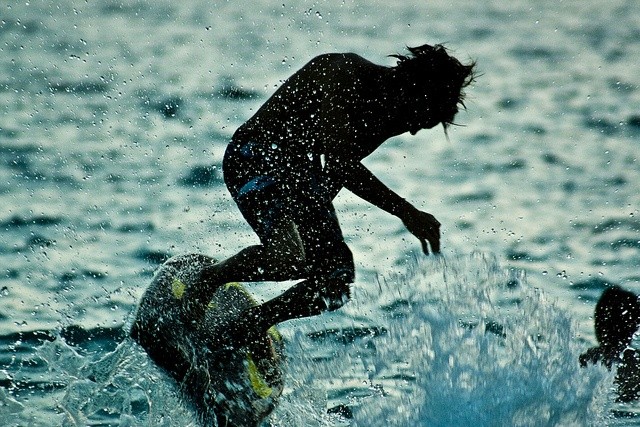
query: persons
185 44 474 356
579 287 640 403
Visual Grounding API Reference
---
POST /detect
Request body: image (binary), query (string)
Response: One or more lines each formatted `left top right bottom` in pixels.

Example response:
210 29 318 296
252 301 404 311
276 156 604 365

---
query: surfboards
129 253 288 427
